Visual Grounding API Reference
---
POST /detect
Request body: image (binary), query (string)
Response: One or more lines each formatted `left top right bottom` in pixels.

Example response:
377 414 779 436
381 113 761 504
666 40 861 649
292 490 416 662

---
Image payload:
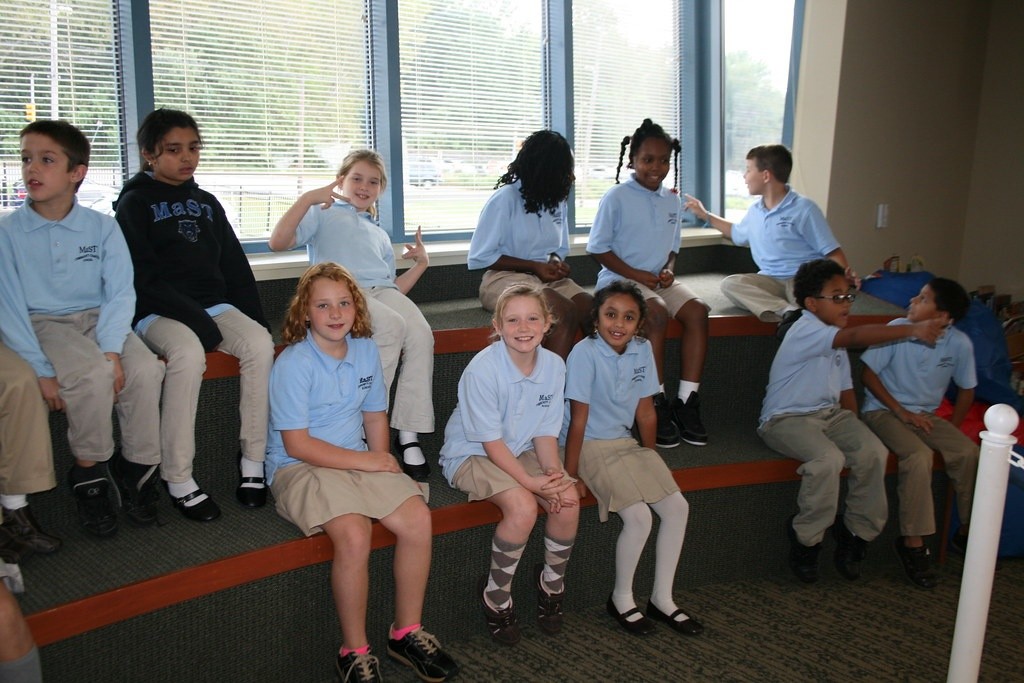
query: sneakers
333 644 385 683
536 569 564 633
386 623 456 682
481 589 522 644
650 391 708 448
69 464 117 537
118 457 168 524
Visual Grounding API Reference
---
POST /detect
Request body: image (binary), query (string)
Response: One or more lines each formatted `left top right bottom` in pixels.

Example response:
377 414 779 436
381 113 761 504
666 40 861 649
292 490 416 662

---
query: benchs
12 272 954 683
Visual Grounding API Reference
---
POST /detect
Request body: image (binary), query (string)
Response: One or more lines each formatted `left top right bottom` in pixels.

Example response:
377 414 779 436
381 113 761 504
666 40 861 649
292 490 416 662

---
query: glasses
816 293 857 304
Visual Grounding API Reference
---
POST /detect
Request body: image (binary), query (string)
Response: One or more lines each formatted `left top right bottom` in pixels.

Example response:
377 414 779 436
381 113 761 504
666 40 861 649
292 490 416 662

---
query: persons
0 340 63 565
855 277 981 591
113 108 274 522
0 556 43 683
438 282 580 645
0 119 165 539
559 281 703 637
269 150 436 481
586 118 711 448
264 262 459 683
757 258 948 585
683 144 860 339
468 129 593 364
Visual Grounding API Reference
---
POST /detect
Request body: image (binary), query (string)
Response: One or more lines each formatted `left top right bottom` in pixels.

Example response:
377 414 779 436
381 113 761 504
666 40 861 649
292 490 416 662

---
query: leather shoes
775 307 802 342
951 528 1003 574
788 512 821 584
892 537 938 590
830 518 867 581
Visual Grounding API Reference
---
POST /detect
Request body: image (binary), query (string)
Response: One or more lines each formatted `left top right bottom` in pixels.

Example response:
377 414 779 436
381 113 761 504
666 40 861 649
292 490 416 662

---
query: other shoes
394 434 431 482
646 598 704 634
607 592 656 635
0 503 63 564
165 482 221 522
237 462 267 510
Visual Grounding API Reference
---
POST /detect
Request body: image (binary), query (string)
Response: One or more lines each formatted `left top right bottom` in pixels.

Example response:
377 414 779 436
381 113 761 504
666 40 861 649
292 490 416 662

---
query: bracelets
704 210 711 227
894 406 903 414
